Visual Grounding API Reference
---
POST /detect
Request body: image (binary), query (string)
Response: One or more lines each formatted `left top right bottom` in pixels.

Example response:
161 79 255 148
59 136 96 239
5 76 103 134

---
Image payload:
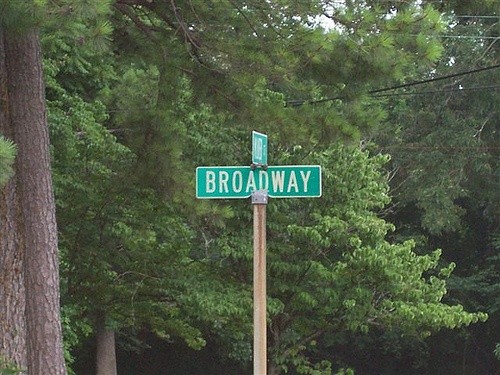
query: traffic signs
195 164 323 200
251 131 268 165
